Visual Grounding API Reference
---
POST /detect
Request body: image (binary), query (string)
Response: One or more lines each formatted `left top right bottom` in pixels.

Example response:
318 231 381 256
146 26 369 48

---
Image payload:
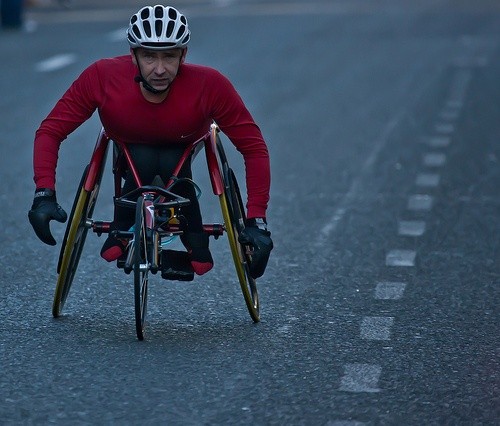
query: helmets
125 5 191 50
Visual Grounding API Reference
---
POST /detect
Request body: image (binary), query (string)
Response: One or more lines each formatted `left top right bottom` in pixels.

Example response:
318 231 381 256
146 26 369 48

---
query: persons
28 5 274 279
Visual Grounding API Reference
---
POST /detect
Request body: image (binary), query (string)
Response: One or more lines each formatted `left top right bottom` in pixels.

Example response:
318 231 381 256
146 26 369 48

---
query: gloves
238 217 273 280
28 188 67 246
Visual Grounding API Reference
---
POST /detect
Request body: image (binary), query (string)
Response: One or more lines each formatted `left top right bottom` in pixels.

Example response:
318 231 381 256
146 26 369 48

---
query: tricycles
52 125 260 341
